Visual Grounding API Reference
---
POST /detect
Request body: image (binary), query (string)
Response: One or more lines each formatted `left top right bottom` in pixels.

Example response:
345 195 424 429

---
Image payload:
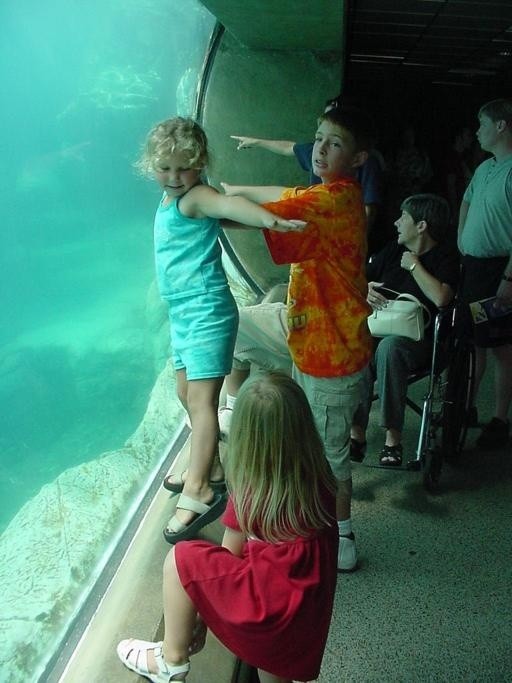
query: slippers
164 469 227 494
163 494 226 544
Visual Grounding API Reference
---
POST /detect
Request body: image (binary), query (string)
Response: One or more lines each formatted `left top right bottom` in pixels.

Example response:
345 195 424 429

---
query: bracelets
409 260 419 273
501 274 511 282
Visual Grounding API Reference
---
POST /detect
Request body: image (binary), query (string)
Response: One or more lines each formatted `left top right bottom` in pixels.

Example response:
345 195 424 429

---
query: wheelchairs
350 271 476 472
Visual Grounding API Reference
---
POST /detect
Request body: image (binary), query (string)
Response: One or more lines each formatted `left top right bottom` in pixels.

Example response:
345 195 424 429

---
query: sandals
379 444 403 466
116 638 190 683
349 440 367 462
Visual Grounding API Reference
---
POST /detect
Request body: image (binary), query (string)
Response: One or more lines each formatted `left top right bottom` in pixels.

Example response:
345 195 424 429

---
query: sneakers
183 405 234 442
473 418 511 452
466 408 478 428
338 532 357 571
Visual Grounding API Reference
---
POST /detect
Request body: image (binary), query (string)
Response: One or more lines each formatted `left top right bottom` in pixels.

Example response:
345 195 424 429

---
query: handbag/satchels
367 293 431 342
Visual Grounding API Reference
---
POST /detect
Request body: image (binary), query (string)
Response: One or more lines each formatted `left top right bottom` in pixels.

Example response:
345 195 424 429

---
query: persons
230 87 388 285
453 98 512 455
146 113 314 546
117 368 346 682
222 98 375 573
344 187 465 472
